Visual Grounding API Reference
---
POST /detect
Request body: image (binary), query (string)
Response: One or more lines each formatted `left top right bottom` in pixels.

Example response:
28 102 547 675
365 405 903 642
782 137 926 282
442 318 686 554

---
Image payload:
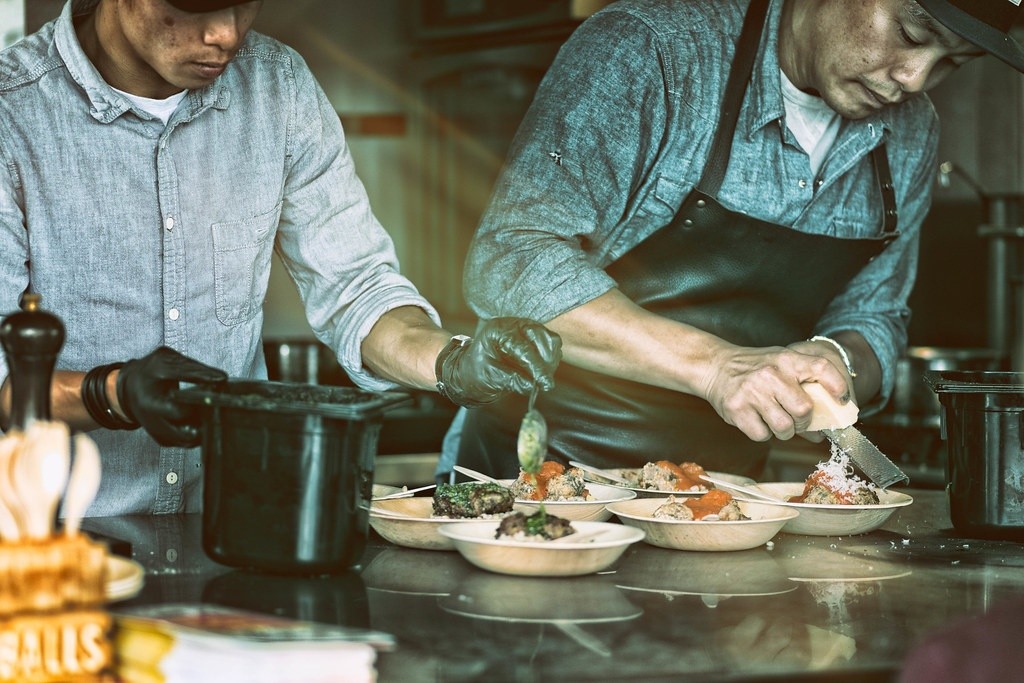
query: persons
434 0 1024 491
0 0 561 521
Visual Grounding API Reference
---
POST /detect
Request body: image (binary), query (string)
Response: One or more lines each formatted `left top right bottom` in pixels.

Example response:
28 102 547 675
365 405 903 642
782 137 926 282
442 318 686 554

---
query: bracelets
81 361 143 432
807 335 857 382
435 333 471 406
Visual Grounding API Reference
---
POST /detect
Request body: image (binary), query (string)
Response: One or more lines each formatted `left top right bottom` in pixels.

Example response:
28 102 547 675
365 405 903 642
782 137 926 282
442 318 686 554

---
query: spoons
517 384 547 474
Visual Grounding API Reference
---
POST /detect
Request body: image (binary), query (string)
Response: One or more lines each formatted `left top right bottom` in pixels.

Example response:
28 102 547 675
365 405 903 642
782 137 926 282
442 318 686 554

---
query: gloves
440 314 564 409
114 344 229 448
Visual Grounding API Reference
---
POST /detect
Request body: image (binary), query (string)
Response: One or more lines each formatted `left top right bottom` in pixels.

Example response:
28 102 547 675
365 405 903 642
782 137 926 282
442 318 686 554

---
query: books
107 604 396 683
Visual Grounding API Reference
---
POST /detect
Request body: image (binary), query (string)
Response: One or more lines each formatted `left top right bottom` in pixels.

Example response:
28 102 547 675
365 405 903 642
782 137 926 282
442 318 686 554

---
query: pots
182 383 423 578
922 371 1023 541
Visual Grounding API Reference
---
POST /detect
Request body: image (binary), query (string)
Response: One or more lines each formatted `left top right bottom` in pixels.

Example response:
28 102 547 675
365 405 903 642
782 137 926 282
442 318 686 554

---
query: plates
439 520 646 575
459 478 638 521
368 496 540 550
371 485 414 500
607 498 800 551
584 468 756 498
758 482 914 536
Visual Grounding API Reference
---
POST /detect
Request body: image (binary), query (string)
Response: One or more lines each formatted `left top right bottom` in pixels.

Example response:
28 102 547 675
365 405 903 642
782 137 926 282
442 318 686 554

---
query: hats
919 0 1024 75
